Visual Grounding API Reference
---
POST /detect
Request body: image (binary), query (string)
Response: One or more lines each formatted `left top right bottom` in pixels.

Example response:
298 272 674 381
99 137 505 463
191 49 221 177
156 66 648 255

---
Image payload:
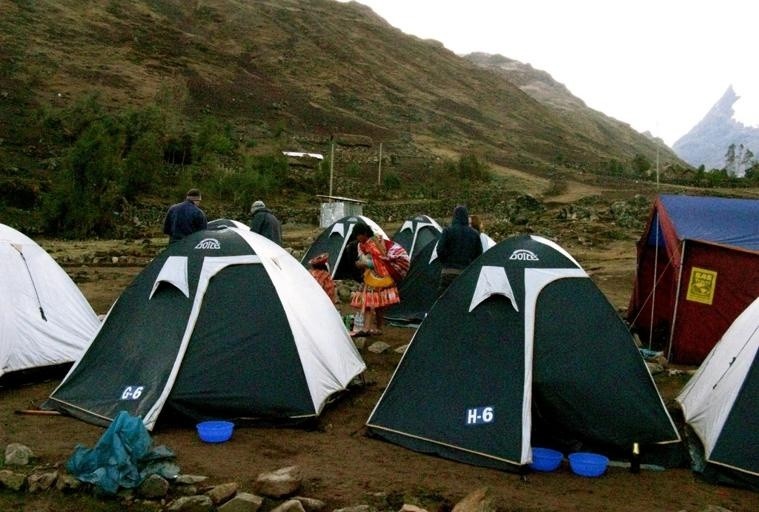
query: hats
311 252 330 265
186 189 202 202
251 200 265 207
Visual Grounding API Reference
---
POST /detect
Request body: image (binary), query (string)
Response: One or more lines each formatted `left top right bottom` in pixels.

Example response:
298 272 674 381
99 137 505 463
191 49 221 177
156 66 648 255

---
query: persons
436 206 483 296
163 188 208 245
352 222 400 336
248 200 282 247
307 253 337 304
469 213 497 256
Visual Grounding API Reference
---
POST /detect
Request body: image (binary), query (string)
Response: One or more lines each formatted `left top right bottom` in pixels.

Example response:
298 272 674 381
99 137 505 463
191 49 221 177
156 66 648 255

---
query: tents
301 215 391 283
673 296 759 492
207 218 250 230
0 222 101 389
627 194 759 364
35 223 368 439
366 233 684 476
373 214 451 326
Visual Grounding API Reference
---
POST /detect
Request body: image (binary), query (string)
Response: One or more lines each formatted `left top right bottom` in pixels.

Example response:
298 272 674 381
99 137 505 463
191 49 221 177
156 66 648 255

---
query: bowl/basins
529 448 562 473
568 453 608 478
196 421 234 444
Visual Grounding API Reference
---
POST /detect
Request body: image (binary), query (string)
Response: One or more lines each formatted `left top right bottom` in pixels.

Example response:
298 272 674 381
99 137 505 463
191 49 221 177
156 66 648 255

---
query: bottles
687 444 700 464
631 442 640 473
345 311 364 332
